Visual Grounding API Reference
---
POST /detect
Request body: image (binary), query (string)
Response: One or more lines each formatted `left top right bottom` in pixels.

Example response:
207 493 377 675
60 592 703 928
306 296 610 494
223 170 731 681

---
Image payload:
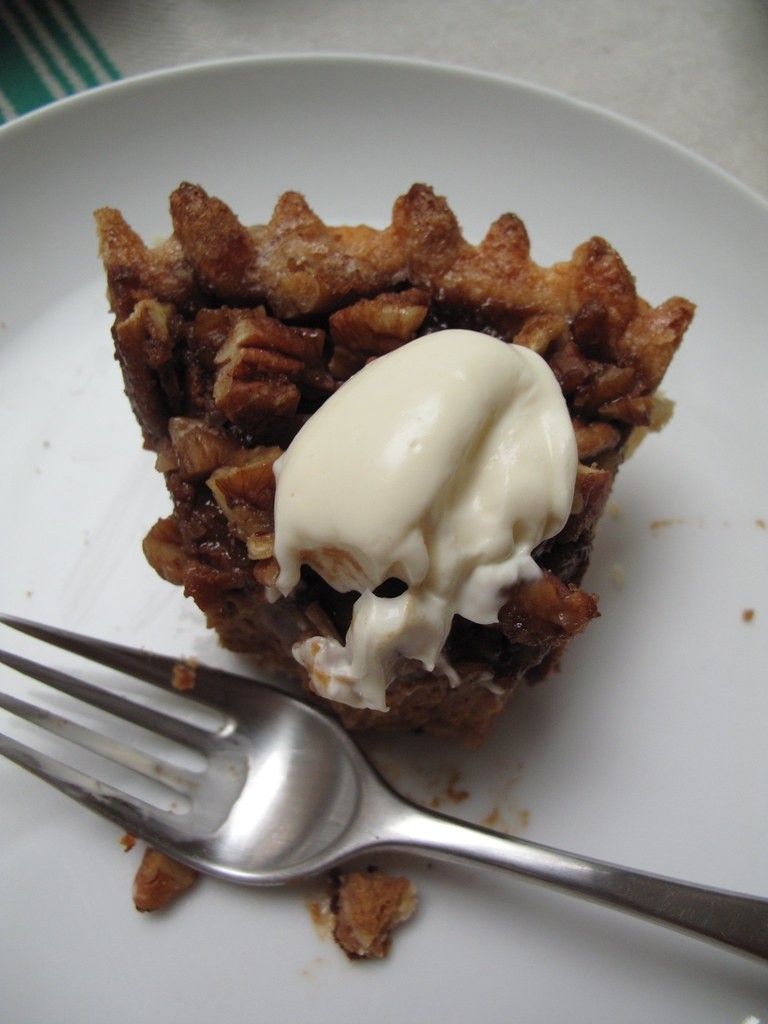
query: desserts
93 182 699 738
